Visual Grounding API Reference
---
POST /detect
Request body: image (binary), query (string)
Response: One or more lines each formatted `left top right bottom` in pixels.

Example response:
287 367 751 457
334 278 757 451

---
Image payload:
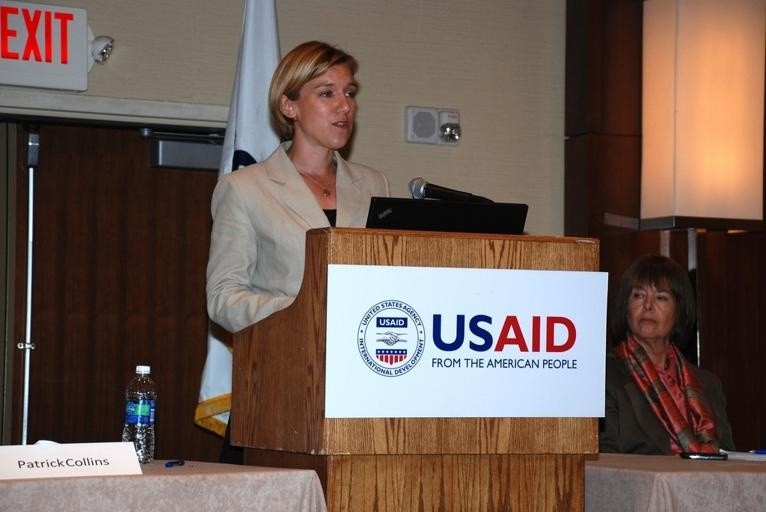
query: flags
192 1 279 444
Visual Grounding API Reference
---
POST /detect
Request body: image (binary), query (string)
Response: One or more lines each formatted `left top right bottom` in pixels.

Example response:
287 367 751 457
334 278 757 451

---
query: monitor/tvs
365 196 529 233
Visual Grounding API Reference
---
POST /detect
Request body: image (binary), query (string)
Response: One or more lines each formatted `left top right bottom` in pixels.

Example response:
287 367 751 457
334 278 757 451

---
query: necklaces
296 162 334 198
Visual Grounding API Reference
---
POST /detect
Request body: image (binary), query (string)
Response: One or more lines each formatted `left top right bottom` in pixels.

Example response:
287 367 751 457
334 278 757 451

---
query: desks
0 458 321 511
585 452 766 511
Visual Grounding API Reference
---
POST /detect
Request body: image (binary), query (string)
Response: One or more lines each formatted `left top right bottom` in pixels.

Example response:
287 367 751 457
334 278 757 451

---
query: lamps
638 1 766 232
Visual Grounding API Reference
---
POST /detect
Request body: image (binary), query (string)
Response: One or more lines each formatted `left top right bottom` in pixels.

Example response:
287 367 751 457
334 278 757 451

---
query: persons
207 39 389 469
601 253 738 454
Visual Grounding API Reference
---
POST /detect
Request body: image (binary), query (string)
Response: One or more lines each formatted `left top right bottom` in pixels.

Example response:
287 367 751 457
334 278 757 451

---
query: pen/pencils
748 449 766 454
165 460 185 467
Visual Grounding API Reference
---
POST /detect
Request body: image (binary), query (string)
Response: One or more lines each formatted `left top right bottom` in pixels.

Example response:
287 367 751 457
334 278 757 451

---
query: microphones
406 177 493 202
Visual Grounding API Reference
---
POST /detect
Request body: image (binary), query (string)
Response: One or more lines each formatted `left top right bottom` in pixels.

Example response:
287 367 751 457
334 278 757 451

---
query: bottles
123 366 157 463
